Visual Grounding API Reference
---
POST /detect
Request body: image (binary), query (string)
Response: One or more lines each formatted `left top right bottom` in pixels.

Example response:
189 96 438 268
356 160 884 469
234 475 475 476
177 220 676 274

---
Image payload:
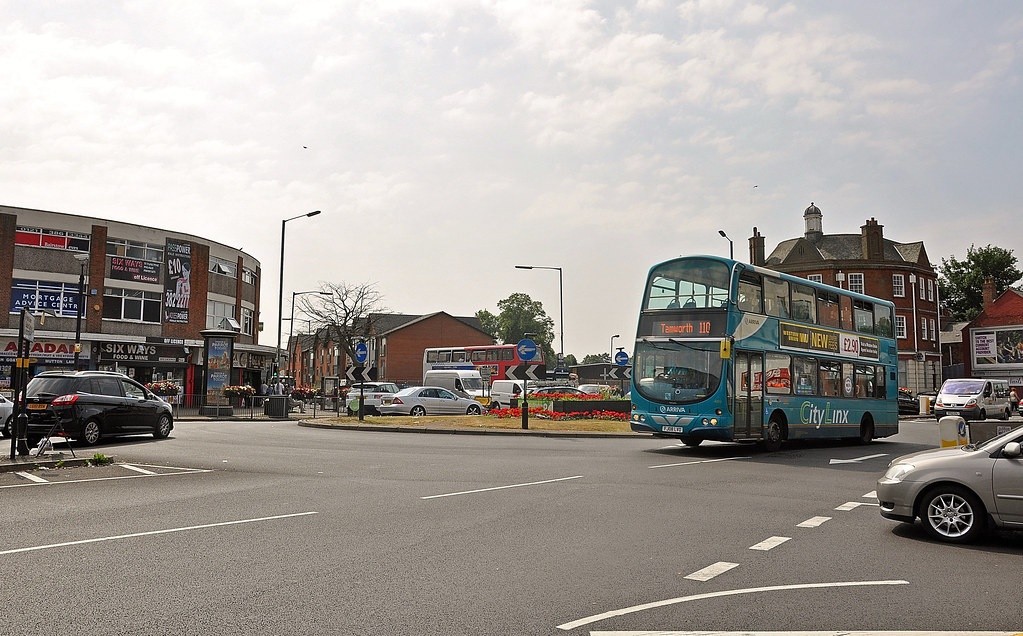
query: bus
629 255 899 450
422 345 546 388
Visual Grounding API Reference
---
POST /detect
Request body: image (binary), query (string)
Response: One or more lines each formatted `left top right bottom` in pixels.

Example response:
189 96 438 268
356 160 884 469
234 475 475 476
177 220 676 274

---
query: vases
154 391 178 396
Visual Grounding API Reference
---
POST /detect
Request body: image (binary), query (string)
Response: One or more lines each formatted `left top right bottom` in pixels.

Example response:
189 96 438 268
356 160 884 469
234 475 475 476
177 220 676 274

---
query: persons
821 372 875 398
261 379 306 413
996 338 1023 363
174 262 190 306
1010 387 1020 411
663 356 690 388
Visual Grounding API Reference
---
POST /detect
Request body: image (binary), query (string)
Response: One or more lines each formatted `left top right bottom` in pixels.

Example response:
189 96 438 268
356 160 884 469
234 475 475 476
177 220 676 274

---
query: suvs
522 385 588 395
577 384 610 395
14 370 174 445
346 382 400 415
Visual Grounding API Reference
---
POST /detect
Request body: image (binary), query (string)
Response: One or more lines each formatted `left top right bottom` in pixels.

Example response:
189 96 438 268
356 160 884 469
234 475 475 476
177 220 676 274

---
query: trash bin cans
269 395 289 418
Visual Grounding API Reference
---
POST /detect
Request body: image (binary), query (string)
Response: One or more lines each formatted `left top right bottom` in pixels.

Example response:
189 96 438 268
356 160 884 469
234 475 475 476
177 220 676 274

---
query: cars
918 391 938 409
898 392 920 415
379 387 482 417
0 394 14 439
875 425 1023 545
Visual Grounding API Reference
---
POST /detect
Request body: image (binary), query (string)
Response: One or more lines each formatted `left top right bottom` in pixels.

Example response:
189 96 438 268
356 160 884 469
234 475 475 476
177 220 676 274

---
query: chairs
721 296 751 311
683 298 696 307
667 298 680 308
288 400 301 413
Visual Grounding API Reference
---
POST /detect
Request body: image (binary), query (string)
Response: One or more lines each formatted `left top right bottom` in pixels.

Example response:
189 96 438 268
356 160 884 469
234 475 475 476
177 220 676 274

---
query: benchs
474 396 492 411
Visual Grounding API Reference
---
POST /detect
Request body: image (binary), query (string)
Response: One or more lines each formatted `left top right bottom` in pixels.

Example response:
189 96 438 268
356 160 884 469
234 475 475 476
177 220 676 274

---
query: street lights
611 335 619 370
288 291 333 387
719 230 733 259
276 211 321 395
283 318 310 375
515 266 563 353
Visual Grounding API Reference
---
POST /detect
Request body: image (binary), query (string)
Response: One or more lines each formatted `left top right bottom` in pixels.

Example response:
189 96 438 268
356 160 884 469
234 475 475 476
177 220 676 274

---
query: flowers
224 385 255 398
145 381 181 394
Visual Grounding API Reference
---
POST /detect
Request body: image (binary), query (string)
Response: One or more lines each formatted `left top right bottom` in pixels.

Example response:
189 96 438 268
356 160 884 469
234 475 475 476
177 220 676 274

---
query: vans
491 380 538 410
424 370 487 399
933 379 1012 422
